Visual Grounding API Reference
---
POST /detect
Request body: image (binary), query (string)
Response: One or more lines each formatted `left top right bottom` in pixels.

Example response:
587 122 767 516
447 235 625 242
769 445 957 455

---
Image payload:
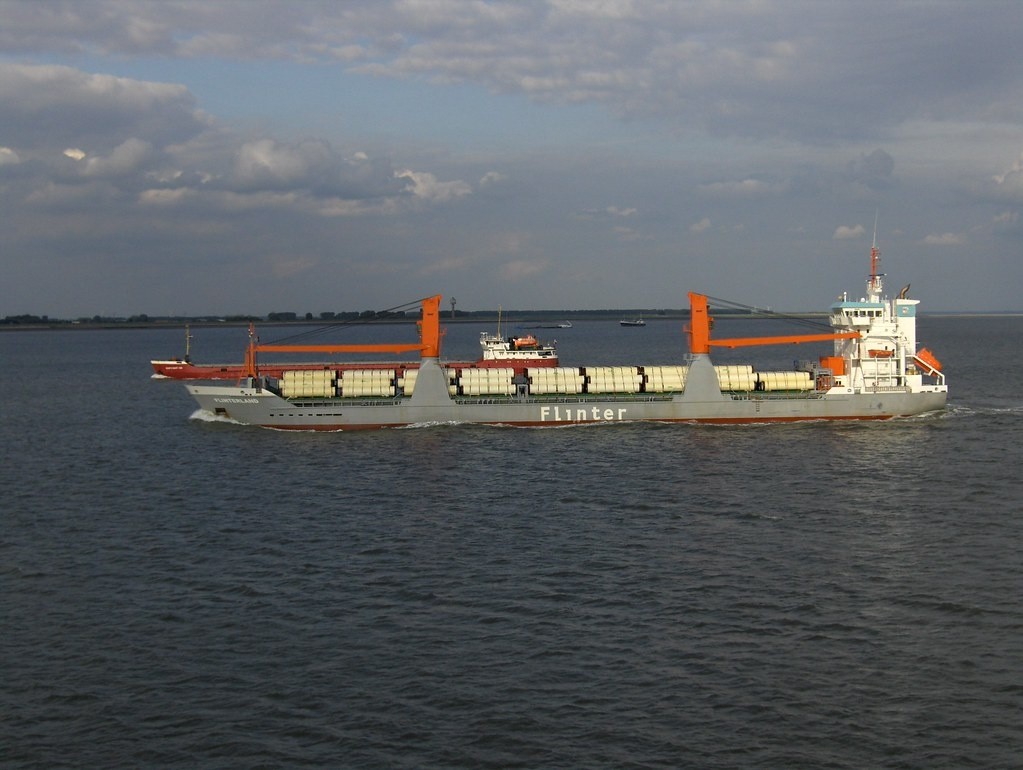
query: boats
151 331 561 381
619 319 647 327
514 320 573 329
178 203 949 434
